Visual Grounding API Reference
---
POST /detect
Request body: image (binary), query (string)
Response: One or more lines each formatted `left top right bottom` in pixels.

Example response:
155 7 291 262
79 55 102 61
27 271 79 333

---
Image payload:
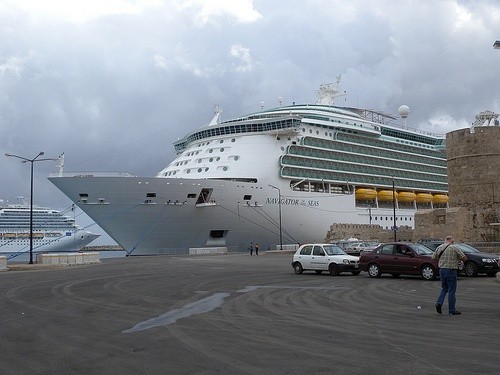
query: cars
358 241 464 281
291 244 362 277
337 240 389 256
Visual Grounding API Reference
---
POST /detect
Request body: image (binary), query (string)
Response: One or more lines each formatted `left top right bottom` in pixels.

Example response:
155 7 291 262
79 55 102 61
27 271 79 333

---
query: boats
3 233 16 239
377 190 398 202
416 193 433 204
397 192 416 203
32 234 44 239
355 188 377 201
433 194 449 205
17 233 30 239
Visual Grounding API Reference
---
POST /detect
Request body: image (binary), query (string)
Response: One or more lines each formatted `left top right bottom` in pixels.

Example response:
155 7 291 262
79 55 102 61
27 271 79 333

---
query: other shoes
449 310 462 315
435 303 442 313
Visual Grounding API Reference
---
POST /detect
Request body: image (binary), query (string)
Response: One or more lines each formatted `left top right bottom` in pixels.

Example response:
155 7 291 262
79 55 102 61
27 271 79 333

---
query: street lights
267 184 283 250
389 173 397 242
361 203 372 241
4 152 59 265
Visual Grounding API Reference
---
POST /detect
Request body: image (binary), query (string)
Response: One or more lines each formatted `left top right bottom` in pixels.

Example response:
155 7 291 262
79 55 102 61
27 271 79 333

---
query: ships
0 195 102 254
46 73 450 257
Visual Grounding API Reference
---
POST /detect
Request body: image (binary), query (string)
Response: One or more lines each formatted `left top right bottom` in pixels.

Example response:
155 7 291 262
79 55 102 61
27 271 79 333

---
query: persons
432 236 467 316
255 243 259 255
250 242 254 256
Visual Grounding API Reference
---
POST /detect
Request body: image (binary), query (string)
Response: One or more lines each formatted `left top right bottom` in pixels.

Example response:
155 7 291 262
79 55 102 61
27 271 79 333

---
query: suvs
416 237 500 278
